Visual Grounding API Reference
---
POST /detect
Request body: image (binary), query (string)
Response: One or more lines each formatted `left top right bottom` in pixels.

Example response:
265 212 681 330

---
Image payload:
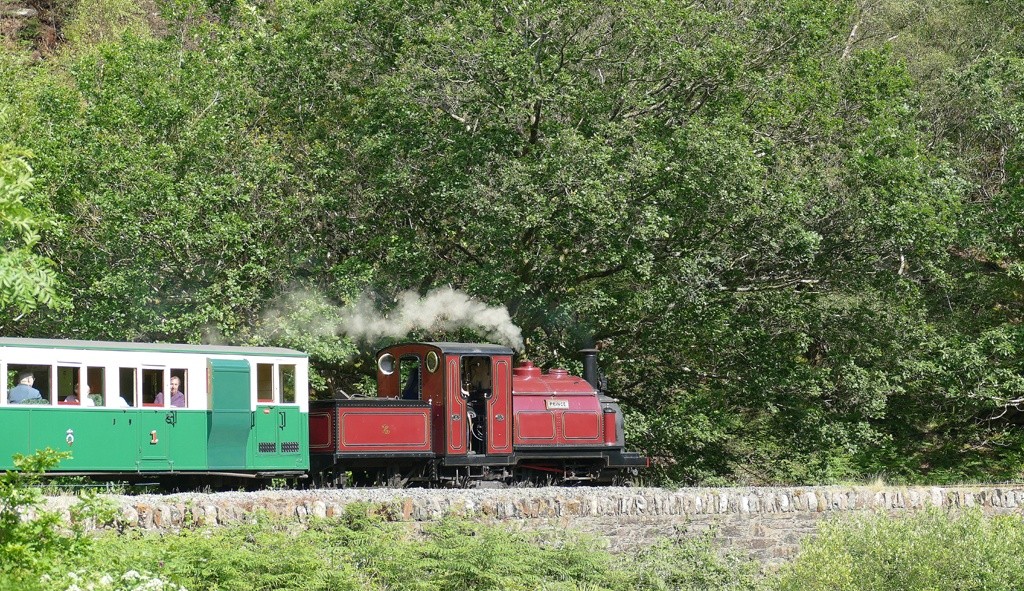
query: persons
8 372 42 404
64 382 94 407
154 376 185 407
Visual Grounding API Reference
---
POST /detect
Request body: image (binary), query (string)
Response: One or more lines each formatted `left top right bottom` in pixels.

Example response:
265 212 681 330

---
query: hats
17 371 33 383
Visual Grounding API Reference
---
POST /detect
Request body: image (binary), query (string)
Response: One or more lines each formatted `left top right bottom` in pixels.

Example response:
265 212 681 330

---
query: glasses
33 377 35 381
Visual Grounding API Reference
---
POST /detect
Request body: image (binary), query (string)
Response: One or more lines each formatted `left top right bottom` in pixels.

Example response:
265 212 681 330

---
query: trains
0 337 649 493
